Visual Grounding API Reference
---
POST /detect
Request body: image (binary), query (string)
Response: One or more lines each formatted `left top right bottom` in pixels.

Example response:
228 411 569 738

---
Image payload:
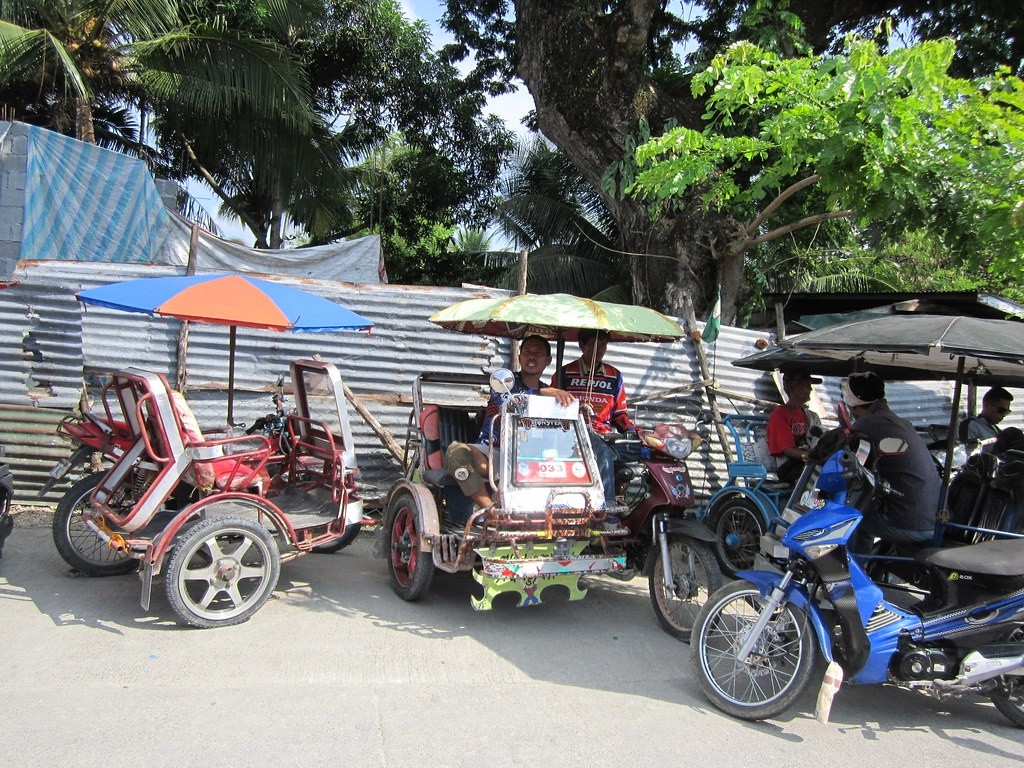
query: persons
445 334 577 519
959 387 1014 453
767 374 822 502
550 328 647 513
811 371 955 574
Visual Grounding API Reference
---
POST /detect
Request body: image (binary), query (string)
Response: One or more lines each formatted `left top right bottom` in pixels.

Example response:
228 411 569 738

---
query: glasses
990 403 1012 417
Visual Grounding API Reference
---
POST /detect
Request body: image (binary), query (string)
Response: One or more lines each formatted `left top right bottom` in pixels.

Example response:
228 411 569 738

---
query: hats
782 370 824 384
844 371 885 406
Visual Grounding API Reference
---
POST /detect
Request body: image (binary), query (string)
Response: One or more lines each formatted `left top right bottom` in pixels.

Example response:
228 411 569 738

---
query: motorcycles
690 314 1024 729
381 292 722 643
35 272 378 629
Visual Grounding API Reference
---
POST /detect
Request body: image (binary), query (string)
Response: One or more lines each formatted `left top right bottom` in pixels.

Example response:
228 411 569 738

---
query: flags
701 293 720 343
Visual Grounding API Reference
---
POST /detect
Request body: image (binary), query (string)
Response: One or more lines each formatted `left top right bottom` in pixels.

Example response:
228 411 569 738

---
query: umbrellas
730 313 1024 545
427 293 688 389
76 271 375 423
